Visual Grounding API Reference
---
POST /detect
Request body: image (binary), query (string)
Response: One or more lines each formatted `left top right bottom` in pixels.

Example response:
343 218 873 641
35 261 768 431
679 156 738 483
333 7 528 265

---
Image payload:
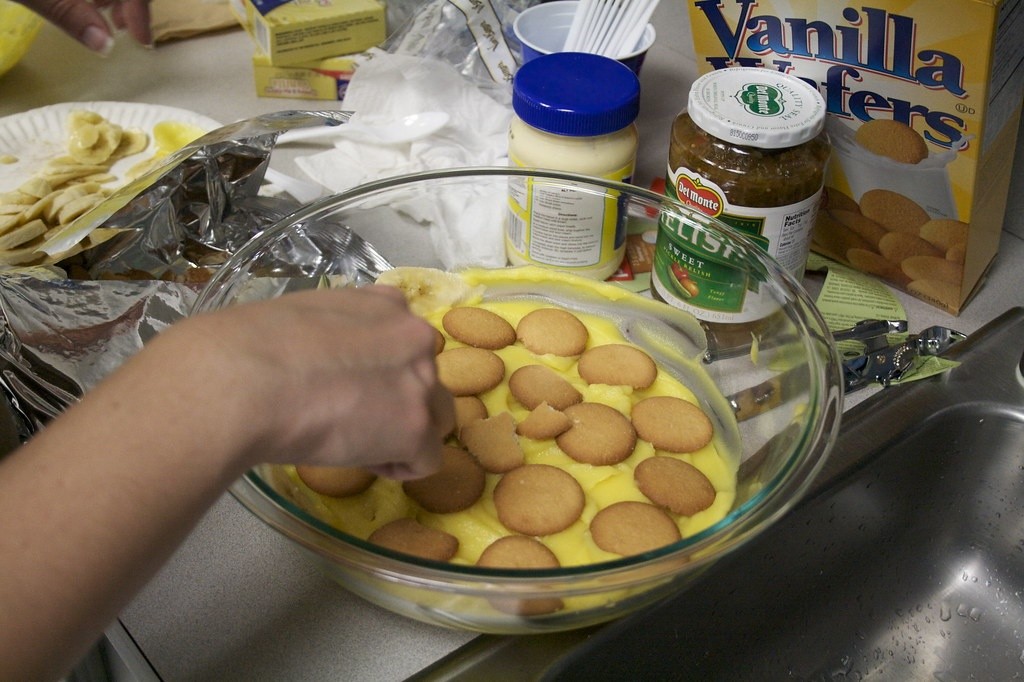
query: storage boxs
226 0 387 67
252 44 362 100
687 0 1024 316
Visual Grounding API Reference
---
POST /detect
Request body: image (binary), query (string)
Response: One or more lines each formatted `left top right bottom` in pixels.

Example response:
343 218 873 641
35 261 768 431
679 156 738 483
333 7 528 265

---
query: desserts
268 298 742 629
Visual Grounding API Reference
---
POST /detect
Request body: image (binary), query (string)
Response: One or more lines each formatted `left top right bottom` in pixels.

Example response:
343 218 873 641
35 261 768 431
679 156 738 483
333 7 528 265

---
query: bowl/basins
189 166 843 634
0 1 47 75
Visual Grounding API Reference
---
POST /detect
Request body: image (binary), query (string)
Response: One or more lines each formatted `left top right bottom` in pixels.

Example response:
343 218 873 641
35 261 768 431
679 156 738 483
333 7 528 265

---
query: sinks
401 398 1024 682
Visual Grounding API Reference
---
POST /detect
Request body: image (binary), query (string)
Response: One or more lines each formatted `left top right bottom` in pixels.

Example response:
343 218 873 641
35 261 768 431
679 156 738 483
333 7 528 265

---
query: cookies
809 119 969 314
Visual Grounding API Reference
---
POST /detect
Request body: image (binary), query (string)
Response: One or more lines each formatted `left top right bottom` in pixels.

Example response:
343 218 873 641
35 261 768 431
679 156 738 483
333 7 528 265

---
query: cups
512 1 657 78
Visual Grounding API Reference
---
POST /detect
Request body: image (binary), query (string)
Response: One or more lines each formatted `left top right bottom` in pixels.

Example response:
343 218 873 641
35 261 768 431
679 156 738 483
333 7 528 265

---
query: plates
0 101 224 190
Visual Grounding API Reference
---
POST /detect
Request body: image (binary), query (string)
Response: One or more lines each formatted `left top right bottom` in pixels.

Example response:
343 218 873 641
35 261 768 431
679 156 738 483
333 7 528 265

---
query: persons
0 0 456 682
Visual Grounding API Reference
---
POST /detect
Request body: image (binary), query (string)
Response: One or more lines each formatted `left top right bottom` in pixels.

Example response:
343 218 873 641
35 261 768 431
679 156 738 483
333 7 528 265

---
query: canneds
502 52 642 284
649 65 830 349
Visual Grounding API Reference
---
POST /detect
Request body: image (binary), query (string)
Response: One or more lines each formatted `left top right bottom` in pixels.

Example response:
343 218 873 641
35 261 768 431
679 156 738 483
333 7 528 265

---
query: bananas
1 111 199 268
